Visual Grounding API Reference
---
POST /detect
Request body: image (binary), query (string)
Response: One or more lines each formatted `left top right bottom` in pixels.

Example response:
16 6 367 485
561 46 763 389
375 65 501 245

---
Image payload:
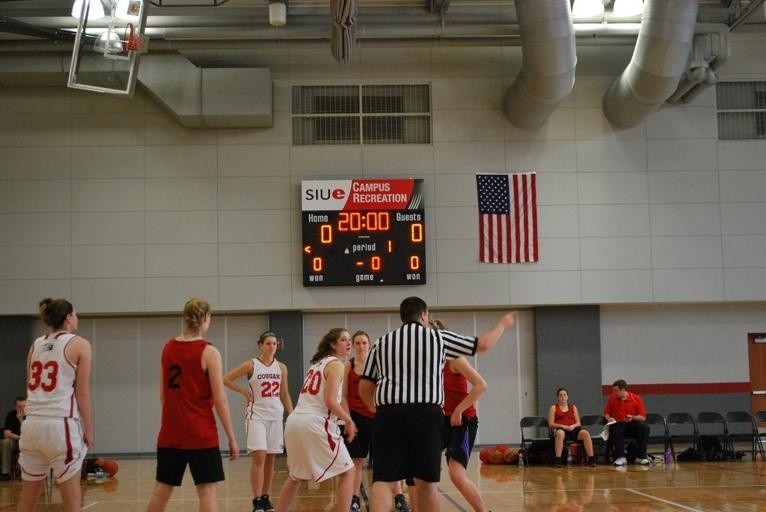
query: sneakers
262 495 275 512
352 495 360 512
395 494 411 512
613 457 627 466
635 457 649 465
253 495 265 512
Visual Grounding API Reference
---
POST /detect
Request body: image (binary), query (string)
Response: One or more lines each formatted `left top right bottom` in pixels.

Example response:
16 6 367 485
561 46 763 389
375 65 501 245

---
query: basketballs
480 445 519 465
94 457 118 476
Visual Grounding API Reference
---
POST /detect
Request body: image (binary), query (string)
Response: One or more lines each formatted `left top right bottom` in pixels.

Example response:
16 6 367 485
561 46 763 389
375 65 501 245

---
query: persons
603 378 652 467
358 294 518 511
343 333 407 511
429 319 490 512
16 298 94 512
1 396 26 482
146 298 239 511
274 327 360 511
223 328 294 511
547 388 597 468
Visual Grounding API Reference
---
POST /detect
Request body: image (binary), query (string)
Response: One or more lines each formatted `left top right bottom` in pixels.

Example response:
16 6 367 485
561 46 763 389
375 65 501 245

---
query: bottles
95 466 103 485
87 473 112 482
519 452 523 466
666 448 670 463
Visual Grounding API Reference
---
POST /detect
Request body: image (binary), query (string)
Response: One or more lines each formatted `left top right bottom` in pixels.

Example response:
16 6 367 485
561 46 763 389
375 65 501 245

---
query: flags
475 170 541 266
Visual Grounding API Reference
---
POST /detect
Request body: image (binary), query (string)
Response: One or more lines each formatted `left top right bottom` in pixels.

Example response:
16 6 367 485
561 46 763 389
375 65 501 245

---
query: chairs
519 410 766 464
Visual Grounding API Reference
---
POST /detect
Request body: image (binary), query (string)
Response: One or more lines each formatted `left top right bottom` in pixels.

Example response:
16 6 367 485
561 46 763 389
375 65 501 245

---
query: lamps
92 21 123 54
